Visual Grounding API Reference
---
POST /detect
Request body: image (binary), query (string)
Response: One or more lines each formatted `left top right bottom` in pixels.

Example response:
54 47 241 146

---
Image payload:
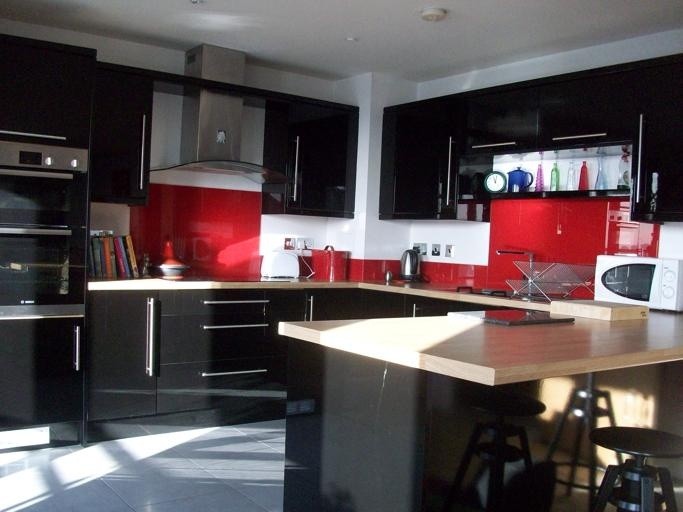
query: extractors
149 41 285 187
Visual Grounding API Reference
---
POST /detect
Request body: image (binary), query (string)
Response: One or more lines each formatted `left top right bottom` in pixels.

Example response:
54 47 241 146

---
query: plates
149 264 192 278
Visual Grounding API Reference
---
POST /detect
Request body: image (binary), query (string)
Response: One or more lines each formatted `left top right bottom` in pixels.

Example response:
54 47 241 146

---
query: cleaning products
578 161 589 190
616 154 631 190
565 160 577 191
535 163 543 192
550 163 560 192
594 157 607 190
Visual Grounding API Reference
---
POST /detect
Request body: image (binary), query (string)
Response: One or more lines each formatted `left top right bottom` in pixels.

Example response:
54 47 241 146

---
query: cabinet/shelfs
260 88 360 219
157 279 299 434
299 283 359 322
535 61 633 150
85 276 157 442
456 76 540 157
0 305 86 451
0 34 97 150
629 52 683 222
378 84 491 224
359 284 553 322
85 57 156 206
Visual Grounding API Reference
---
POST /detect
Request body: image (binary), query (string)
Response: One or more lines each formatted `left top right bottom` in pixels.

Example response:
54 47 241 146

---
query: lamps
419 7 446 22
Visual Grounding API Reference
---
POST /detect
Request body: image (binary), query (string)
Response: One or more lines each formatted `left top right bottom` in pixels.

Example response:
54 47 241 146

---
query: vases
532 157 629 193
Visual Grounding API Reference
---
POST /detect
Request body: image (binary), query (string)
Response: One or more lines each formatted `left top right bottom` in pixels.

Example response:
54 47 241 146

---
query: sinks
506 296 552 304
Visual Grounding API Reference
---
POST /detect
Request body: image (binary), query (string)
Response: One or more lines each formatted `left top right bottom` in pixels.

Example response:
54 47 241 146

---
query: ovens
0 129 86 315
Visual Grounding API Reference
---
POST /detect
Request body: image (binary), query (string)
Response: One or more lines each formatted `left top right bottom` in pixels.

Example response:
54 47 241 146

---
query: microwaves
594 253 682 314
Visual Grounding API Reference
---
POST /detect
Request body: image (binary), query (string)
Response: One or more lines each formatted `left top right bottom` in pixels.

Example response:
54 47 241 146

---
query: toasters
259 252 299 278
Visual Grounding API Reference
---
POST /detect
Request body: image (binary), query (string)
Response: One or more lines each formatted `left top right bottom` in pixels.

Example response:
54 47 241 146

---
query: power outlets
296 238 314 250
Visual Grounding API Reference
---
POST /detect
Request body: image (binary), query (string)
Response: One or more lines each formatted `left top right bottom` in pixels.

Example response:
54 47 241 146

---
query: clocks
484 172 506 193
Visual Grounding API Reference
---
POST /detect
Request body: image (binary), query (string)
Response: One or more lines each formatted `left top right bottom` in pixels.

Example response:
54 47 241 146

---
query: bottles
533 154 631 192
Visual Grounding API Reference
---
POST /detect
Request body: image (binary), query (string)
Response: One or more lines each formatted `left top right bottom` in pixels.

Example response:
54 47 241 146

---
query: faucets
496 249 535 296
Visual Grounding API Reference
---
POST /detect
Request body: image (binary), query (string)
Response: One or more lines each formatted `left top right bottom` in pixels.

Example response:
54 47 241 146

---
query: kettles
397 248 420 280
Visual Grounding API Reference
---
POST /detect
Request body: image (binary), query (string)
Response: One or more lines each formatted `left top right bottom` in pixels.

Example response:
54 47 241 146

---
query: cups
504 167 534 193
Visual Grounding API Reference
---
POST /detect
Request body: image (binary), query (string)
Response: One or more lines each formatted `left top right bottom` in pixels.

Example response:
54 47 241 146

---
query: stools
587 426 682 511
446 387 546 512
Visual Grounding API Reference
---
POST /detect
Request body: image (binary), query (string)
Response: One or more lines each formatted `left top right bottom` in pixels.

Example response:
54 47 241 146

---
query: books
87 231 141 279
